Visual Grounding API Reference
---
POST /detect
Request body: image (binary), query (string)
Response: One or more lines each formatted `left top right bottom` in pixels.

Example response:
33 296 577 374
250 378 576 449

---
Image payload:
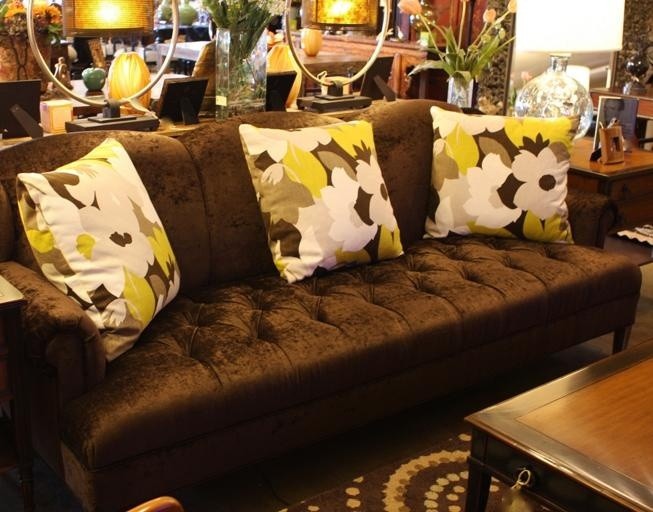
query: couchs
0 99 644 511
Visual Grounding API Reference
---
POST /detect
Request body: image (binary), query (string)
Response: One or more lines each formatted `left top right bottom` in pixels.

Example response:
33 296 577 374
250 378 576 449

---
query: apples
157 7 172 21
180 5 197 25
82 68 105 91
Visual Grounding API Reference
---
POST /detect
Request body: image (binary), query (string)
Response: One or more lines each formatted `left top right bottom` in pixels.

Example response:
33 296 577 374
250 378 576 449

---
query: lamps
285 1 392 113
508 0 623 144
24 0 179 134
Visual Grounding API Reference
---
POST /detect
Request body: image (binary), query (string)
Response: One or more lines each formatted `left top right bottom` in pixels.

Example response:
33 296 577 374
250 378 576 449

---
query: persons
603 99 631 138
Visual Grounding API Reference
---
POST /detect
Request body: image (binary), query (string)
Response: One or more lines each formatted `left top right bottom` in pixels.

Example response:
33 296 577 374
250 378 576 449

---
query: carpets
274 435 513 509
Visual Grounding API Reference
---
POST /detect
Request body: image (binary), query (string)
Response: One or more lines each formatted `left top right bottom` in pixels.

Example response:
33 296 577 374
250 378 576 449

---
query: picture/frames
590 96 640 161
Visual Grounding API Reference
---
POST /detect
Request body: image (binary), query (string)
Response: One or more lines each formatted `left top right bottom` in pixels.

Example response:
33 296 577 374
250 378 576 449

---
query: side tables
566 136 652 249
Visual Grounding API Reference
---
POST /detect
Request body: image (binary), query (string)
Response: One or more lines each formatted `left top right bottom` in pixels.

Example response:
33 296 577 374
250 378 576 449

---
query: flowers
397 0 518 89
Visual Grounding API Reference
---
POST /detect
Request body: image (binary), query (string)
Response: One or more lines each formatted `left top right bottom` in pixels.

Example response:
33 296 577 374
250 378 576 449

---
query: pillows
423 106 580 245
238 119 404 286
15 136 183 363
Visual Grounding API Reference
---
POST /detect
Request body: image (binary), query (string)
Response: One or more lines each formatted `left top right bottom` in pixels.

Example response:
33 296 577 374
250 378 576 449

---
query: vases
444 78 472 110
217 27 268 121
